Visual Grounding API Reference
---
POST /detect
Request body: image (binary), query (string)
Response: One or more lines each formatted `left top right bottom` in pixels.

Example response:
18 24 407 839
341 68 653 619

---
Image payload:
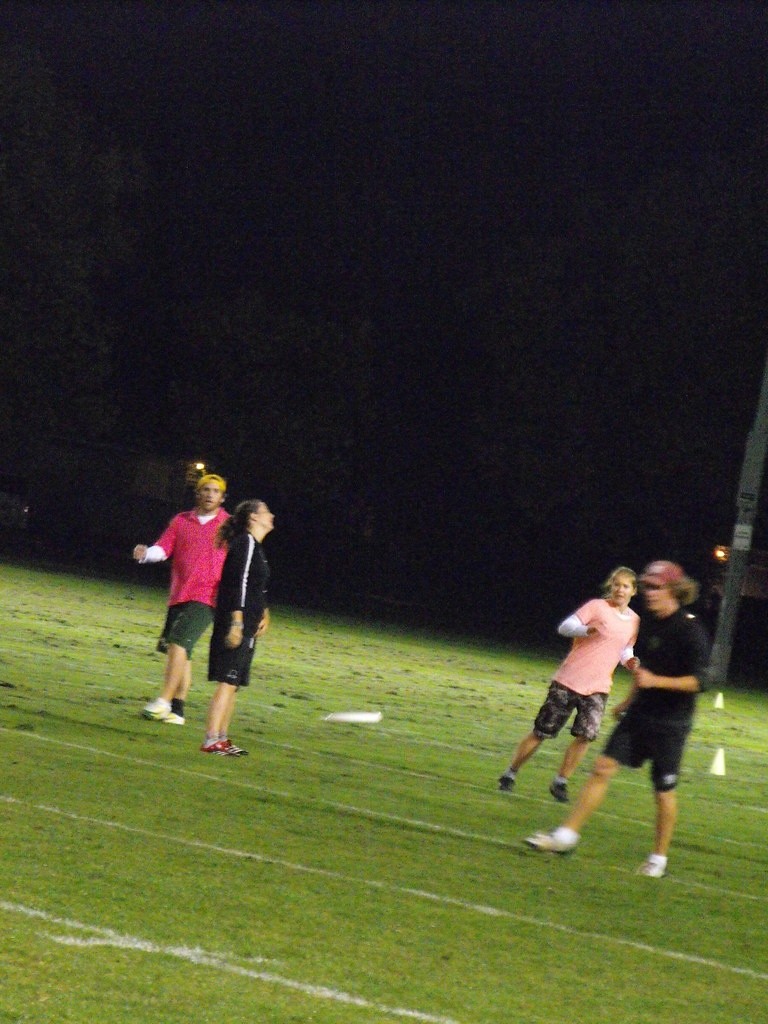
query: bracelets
232 621 245 628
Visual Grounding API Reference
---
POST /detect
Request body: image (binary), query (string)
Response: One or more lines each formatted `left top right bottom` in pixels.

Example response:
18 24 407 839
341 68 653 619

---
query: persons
134 474 236 725
201 500 274 757
499 567 641 804
521 560 714 880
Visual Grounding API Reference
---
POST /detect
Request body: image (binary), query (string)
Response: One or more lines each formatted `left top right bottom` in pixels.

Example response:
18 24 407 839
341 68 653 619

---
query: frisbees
327 712 385 725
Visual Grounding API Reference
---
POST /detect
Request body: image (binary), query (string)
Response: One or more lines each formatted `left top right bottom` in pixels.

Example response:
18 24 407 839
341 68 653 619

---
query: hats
635 560 683 587
197 474 227 492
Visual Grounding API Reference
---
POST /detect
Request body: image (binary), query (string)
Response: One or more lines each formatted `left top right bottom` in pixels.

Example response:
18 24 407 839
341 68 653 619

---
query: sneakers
200 739 249 757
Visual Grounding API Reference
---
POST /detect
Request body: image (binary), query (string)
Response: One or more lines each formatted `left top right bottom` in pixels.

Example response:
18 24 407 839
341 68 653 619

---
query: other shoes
636 858 666 878
498 774 515 792
549 782 570 805
520 831 577 856
142 698 186 725
